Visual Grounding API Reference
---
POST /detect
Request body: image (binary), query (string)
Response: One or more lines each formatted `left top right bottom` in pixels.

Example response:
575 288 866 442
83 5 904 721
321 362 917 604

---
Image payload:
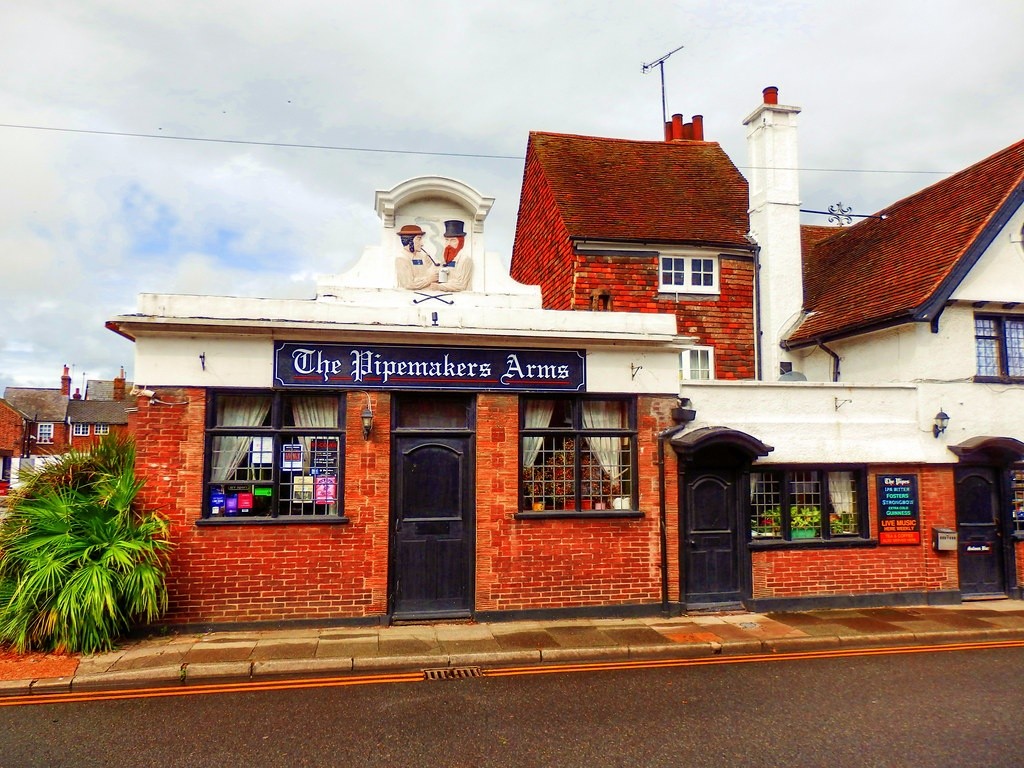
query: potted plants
523 435 631 511
750 504 860 539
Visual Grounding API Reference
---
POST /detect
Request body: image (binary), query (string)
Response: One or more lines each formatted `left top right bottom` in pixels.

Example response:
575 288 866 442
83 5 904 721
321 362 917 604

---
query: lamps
361 403 376 440
933 407 951 438
